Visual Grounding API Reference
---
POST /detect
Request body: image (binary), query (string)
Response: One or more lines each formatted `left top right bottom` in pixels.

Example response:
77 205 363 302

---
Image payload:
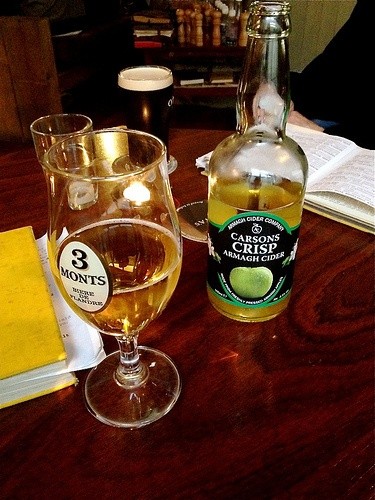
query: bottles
206 1 310 324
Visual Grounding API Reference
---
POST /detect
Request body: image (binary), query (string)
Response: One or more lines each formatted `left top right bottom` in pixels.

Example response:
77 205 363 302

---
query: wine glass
43 127 183 429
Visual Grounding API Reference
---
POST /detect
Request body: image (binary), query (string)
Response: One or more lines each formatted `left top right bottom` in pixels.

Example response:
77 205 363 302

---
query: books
132 9 235 85
196 123 375 236
0 226 80 409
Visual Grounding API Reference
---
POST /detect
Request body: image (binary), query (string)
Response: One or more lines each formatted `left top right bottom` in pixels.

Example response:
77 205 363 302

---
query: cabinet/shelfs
142 23 257 117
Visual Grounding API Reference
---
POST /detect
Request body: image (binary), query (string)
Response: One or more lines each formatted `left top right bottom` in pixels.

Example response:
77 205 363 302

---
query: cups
30 113 98 210
118 65 174 167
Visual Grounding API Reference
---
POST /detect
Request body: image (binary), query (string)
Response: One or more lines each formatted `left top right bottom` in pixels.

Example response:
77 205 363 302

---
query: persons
253 0 375 154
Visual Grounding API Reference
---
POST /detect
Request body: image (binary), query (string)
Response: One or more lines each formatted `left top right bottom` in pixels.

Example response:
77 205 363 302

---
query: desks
0 127 375 500
